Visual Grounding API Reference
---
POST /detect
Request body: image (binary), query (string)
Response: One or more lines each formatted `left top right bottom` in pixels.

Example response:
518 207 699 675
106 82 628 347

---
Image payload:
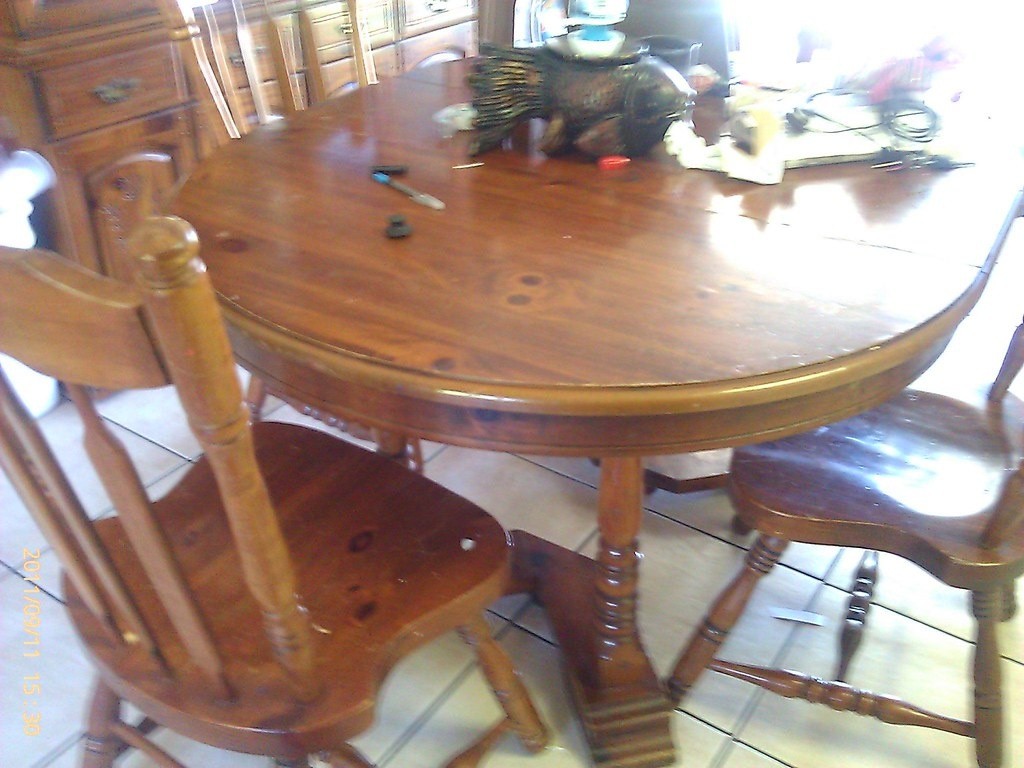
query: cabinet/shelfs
0 0 482 405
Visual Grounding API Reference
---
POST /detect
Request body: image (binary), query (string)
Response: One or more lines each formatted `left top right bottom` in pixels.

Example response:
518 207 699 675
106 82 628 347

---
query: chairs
161 0 428 479
661 317 1024 768
0 215 550 768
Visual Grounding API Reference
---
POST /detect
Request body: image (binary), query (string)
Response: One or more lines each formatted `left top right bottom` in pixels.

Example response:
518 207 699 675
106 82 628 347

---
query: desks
169 46 1024 768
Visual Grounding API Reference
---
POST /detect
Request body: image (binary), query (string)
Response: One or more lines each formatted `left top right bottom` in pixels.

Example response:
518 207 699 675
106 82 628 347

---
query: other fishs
469 42 695 158
